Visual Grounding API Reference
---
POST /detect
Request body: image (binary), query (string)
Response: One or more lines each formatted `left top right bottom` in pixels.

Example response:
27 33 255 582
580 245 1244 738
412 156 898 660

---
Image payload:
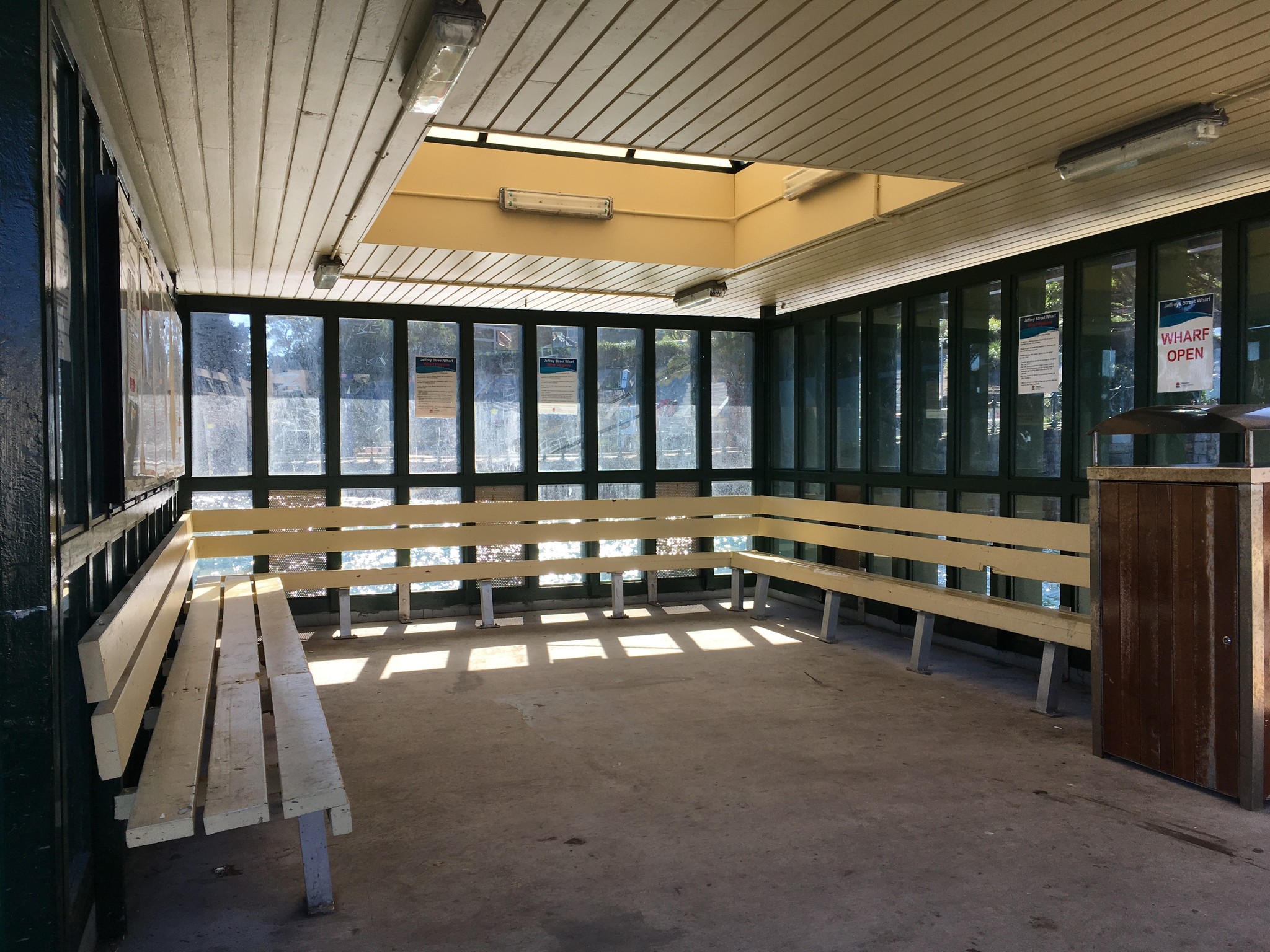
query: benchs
78 510 352 915
735 495 1092 718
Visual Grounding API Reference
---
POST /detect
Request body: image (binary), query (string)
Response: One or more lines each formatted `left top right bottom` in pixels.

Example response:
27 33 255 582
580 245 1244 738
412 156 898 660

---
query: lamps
1053 102 1229 183
782 167 853 201
313 255 343 289
674 280 728 308
499 187 614 221
398 0 486 114
